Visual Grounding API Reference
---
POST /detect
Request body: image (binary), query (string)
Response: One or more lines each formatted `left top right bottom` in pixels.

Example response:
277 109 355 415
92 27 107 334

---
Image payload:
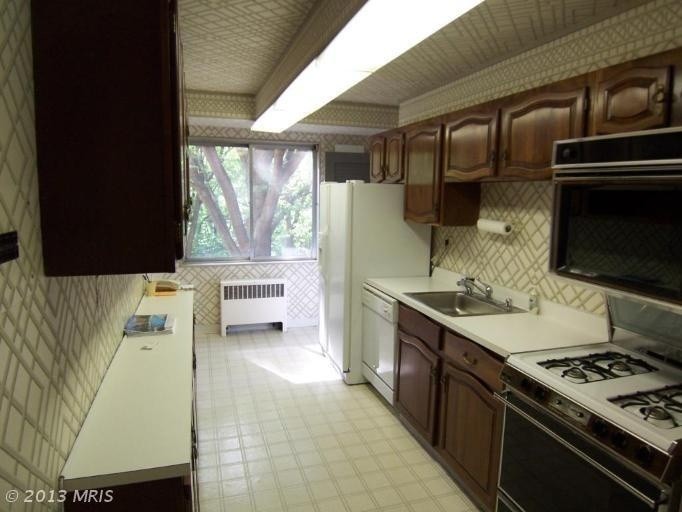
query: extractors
548 127 682 316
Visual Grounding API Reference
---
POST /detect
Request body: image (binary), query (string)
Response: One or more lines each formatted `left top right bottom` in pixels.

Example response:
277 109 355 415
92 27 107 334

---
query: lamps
249 0 483 137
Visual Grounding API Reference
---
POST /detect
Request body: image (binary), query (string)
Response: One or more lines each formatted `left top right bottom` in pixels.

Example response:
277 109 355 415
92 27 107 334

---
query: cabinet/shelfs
51 284 200 512
441 71 587 199
392 304 503 511
363 128 405 184
402 114 480 227
591 48 677 135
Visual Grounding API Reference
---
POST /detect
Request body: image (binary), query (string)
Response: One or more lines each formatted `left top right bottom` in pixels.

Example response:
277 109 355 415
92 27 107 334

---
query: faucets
463 275 493 297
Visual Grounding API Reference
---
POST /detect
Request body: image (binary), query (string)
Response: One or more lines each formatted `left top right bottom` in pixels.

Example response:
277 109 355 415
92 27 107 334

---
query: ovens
494 366 675 511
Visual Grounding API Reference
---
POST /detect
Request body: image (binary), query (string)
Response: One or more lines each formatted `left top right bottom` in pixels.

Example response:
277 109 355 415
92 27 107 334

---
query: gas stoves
500 339 682 454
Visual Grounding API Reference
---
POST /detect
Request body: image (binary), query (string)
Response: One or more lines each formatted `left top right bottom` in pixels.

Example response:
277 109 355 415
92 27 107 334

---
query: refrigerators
316 180 433 386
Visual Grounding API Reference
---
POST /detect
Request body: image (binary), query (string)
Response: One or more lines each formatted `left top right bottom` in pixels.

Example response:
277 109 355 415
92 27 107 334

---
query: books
123 312 176 338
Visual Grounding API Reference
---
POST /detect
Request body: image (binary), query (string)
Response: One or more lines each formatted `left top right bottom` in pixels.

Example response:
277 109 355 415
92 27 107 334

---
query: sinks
403 290 529 318
